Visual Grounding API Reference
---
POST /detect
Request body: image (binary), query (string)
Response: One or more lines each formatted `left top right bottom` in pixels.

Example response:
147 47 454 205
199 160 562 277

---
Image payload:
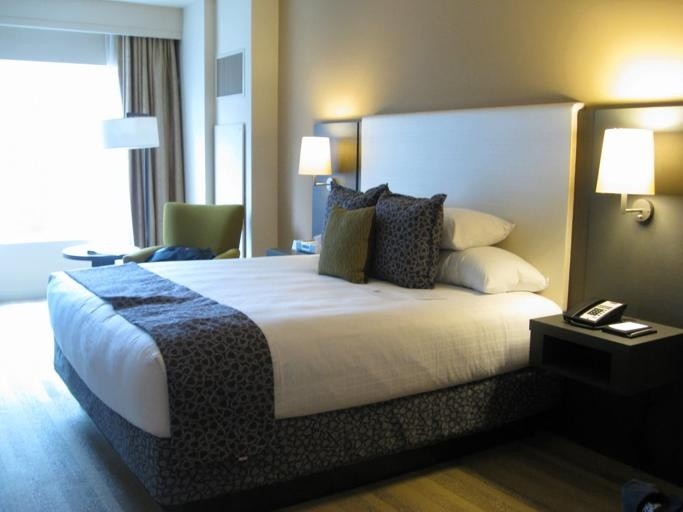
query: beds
52 93 579 512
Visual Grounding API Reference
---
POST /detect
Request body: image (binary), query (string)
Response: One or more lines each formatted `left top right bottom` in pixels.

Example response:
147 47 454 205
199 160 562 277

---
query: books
602 321 657 337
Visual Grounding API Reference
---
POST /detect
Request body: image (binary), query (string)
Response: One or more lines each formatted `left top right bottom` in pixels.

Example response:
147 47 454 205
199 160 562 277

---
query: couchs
124 202 246 266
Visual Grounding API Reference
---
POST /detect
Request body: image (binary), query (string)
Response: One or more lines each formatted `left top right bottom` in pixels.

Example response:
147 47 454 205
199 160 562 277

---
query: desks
62 244 140 268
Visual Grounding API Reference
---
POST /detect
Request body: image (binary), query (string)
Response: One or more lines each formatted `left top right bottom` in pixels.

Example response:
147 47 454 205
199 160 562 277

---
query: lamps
590 125 657 225
101 110 161 248
299 132 336 190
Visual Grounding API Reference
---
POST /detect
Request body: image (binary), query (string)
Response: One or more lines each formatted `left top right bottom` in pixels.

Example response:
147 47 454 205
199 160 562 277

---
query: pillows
426 244 547 293
371 183 446 289
320 180 388 243
317 202 376 284
436 197 517 251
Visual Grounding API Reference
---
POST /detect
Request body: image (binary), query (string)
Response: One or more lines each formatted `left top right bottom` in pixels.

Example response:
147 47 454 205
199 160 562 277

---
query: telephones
563 297 628 326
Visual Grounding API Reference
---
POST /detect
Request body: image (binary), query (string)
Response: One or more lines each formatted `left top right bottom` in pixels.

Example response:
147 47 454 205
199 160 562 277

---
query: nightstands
524 311 681 470
266 247 312 258
